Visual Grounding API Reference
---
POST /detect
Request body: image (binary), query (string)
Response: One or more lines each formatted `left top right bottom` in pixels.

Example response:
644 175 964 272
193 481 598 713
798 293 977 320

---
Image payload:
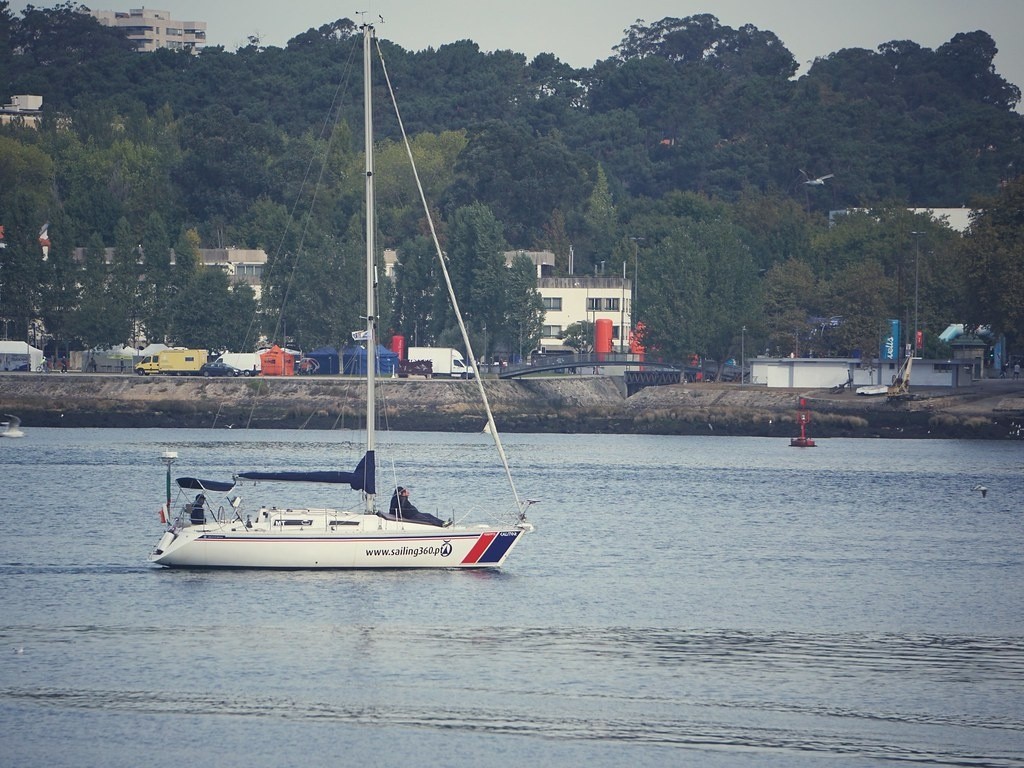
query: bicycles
35 364 52 374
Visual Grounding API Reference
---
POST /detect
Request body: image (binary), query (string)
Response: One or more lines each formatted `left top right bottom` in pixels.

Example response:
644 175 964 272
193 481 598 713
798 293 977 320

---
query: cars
199 362 241 377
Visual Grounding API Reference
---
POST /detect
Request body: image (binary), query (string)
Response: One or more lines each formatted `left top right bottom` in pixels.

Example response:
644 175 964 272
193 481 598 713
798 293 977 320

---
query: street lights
574 282 589 354
413 320 419 347
912 231 925 356
741 326 747 384
518 322 524 380
629 235 645 330
281 319 287 376
576 320 582 376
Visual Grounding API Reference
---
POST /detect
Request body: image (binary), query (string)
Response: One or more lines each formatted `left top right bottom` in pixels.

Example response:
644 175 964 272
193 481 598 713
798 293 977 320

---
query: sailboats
149 9 538 569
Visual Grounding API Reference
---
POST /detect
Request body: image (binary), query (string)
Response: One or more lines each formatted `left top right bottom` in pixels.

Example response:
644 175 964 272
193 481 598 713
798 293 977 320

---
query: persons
191 494 206 523
388 486 453 528
499 358 508 373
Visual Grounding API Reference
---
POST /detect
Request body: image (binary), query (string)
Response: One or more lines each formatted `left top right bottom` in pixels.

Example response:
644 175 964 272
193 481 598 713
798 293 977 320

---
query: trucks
212 350 262 377
406 346 475 379
134 348 207 376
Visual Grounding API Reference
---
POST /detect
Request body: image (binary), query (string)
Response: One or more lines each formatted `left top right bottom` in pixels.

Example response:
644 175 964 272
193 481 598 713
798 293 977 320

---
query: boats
787 409 818 447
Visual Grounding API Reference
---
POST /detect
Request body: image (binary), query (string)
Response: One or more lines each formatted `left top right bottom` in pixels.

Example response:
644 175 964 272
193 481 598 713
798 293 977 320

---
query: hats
393 486 406 496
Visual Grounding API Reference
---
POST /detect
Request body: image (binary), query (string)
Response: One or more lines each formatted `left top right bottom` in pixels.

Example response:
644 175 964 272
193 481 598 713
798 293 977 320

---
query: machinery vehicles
883 349 924 402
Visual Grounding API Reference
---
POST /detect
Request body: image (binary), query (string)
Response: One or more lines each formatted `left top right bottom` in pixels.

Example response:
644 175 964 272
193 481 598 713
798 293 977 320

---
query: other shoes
60 372 62 373
444 517 454 528
65 372 67 374
